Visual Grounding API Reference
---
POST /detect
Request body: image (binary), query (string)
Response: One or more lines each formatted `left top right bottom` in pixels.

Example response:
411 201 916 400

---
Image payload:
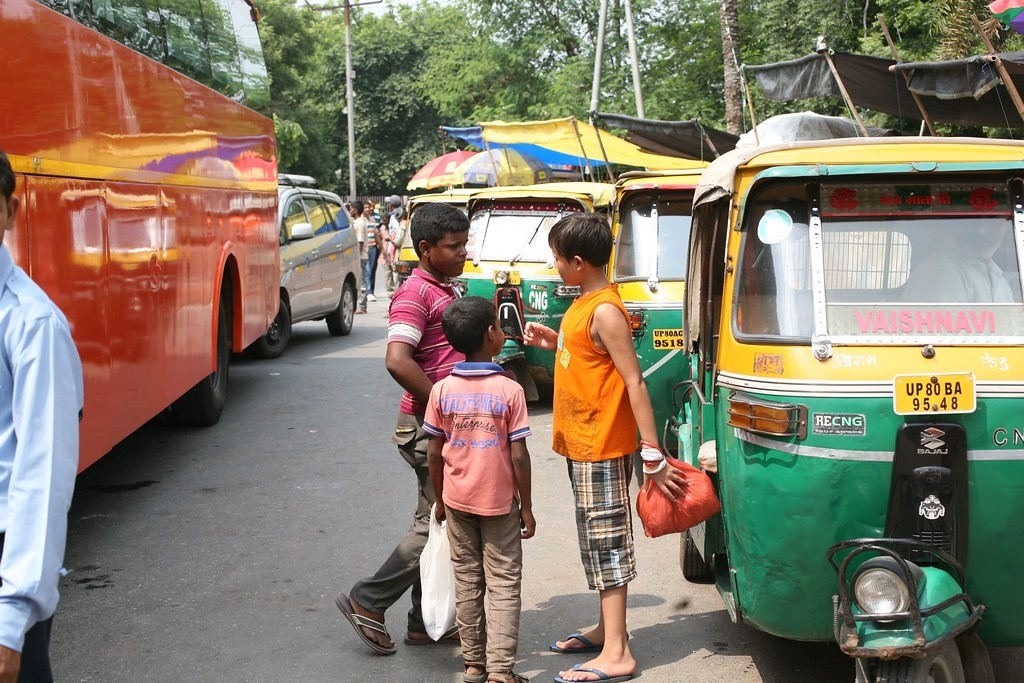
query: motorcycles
686 135 1022 682
592 164 696 471
387 190 477 294
453 187 612 413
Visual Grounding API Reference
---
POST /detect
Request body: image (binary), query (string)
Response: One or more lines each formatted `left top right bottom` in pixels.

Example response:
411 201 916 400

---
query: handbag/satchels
636 439 722 537
420 503 458 642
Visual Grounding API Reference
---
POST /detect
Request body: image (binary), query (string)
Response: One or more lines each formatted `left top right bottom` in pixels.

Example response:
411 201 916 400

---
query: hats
385 195 401 206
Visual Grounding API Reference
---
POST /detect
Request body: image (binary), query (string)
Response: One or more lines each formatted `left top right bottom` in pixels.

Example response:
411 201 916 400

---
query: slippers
404 625 459 644
554 663 633 683
336 592 397 654
550 634 603 653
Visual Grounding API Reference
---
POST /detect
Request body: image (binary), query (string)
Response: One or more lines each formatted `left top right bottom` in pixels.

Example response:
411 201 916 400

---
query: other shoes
355 309 366 314
367 294 377 302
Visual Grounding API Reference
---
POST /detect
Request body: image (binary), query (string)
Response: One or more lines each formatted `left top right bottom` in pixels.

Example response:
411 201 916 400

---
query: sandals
488 671 530 683
463 664 487 683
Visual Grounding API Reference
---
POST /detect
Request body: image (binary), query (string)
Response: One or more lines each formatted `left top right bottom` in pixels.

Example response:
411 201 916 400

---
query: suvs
244 173 364 359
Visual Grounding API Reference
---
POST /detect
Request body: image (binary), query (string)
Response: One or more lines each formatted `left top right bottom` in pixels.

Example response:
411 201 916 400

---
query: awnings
439 107 739 184
735 36 1024 148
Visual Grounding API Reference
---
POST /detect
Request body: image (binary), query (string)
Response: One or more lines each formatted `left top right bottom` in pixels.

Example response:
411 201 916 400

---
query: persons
0 147 84 683
523 213 689 683
336 195 537 683
894 215 1016 304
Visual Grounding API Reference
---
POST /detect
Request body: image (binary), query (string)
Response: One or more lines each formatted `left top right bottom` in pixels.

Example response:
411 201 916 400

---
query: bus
0 0 284 475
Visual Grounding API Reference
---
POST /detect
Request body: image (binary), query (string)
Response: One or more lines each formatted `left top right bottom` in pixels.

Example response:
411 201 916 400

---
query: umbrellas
406 148 553 190
984 0 1024 36
